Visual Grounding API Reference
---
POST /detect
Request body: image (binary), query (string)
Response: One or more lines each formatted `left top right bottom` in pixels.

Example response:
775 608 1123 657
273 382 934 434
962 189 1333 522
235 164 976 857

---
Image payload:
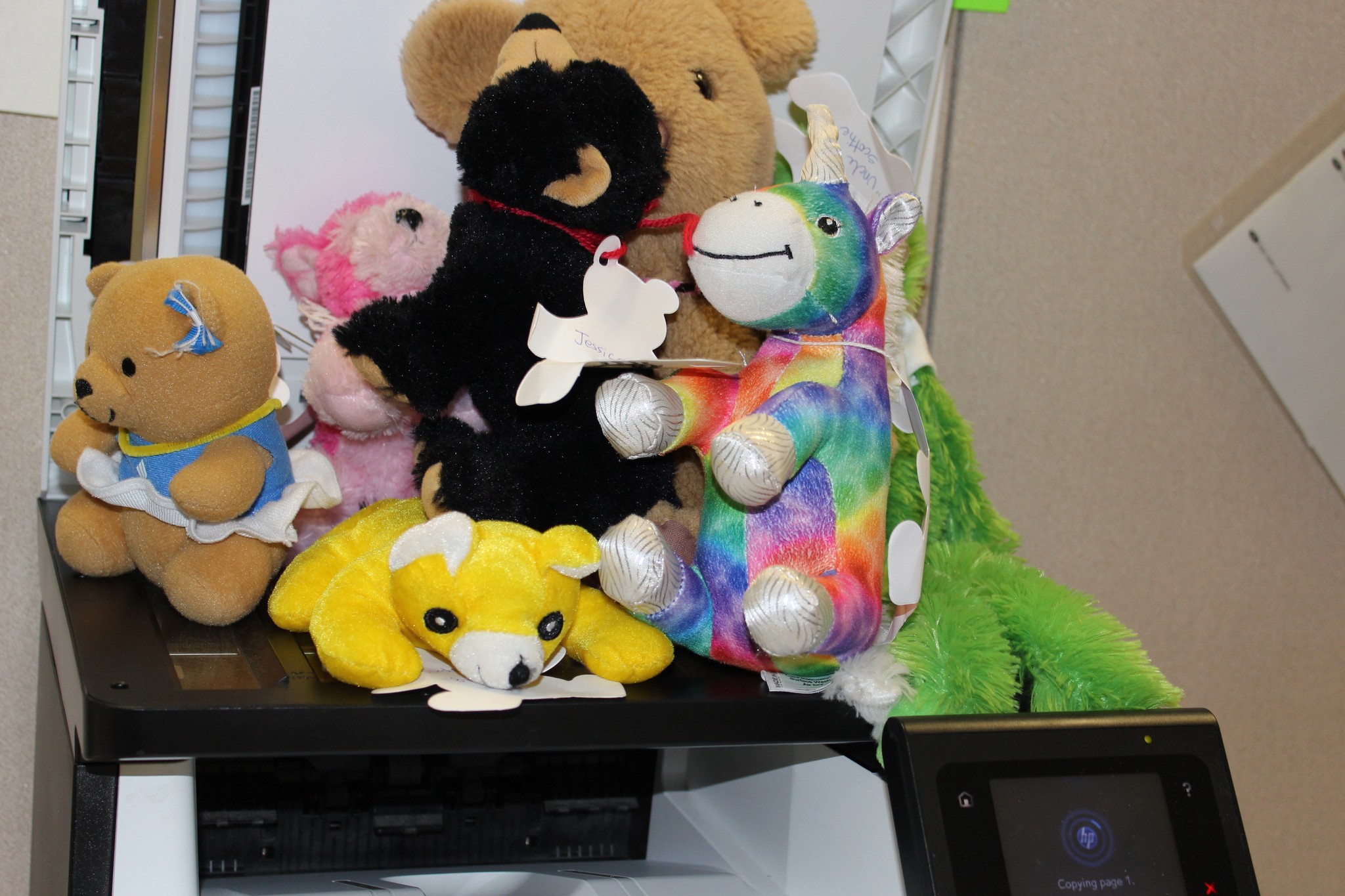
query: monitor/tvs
881 708 1263 896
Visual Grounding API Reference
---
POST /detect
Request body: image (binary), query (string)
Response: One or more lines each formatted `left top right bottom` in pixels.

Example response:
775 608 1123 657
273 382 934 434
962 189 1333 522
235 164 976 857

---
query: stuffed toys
327 9 704 593
400 0 819 572
264 187 448 556
49 254 342 627
266 497 677 692
594 104 926 682
